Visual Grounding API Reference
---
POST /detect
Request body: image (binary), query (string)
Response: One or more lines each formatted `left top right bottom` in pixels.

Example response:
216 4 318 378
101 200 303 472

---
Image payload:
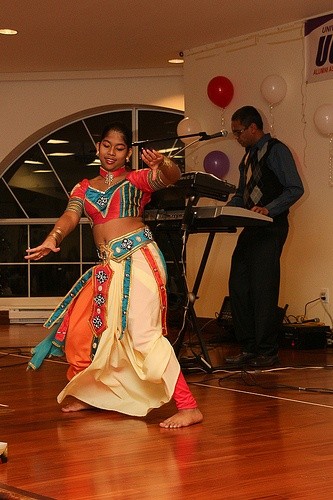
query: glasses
232 121 253 135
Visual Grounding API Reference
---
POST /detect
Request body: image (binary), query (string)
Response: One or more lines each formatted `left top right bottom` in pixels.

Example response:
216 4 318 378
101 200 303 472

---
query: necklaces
100 165 127 185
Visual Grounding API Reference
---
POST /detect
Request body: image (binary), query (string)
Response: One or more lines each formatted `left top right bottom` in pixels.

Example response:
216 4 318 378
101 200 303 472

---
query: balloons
177 117 202 147
261 74 287 105
204 151 230 179
314 104 333 135
207 76 235 110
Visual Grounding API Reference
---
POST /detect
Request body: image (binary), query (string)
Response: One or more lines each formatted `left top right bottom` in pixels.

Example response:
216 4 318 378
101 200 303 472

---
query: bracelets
160 154 171 169
49 232 62 247
52 226 65 240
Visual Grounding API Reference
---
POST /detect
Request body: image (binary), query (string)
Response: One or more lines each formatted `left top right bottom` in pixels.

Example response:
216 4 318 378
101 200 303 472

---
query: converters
196 356 213 374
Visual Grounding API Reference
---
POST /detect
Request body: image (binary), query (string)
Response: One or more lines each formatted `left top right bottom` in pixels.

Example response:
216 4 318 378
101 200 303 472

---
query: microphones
302 317 320 323
199 129 228 141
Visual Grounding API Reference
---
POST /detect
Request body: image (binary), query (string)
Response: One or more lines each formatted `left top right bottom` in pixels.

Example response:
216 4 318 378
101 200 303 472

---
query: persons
24 125 203 429
222 106 304 367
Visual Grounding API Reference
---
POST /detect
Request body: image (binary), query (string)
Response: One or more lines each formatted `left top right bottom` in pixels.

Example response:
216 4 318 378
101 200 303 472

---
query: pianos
140 171 273 376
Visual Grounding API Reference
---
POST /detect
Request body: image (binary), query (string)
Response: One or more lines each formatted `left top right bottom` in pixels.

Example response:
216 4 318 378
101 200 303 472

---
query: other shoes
248 340 285 367
224 351 259 364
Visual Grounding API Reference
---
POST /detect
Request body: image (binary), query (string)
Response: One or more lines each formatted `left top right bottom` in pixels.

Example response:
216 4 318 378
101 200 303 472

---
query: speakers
216 296 285 340
